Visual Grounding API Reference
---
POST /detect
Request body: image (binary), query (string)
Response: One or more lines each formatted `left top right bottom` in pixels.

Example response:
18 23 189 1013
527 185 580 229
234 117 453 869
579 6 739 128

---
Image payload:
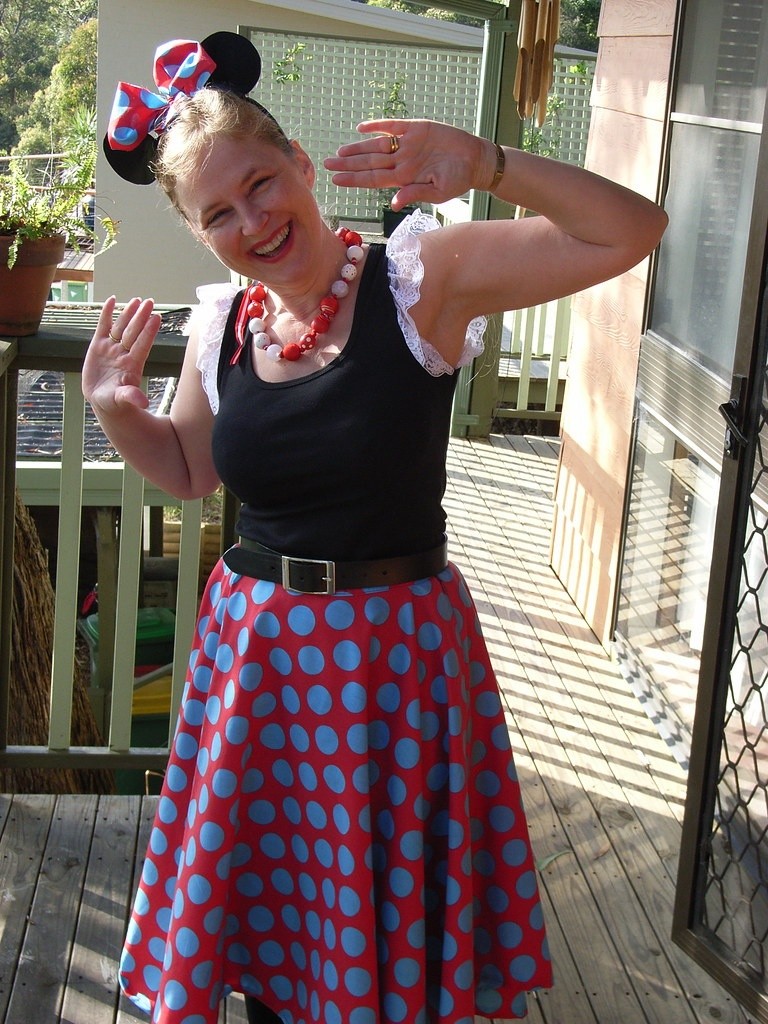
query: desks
15 300 193 691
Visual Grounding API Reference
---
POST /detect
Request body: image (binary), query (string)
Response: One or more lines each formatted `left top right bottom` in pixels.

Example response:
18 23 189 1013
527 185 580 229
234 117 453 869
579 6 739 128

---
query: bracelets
487 142 505 192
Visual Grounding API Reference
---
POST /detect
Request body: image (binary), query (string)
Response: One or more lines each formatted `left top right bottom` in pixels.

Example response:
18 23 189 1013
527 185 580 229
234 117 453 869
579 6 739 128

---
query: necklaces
247 226 365 361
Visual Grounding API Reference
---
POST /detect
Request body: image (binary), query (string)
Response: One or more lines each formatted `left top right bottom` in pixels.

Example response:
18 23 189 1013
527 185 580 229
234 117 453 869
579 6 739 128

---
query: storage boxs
142 557 176 608
77 605 175 746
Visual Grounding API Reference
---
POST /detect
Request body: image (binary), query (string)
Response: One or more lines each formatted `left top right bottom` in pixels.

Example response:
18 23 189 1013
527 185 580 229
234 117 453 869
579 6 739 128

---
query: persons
83 30 670 1023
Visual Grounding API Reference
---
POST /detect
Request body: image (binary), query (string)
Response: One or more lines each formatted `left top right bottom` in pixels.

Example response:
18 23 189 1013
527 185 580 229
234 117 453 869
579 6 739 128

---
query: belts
223 534 446 595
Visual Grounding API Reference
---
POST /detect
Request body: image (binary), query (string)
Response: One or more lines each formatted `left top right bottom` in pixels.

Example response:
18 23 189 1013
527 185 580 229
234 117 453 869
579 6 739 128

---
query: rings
108 333 120 342
389 136 400 153
120 340 131 351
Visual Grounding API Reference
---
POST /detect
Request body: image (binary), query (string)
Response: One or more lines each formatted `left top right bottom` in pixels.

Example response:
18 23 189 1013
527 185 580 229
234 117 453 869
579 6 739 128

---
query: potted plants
0 140 120 337
366 82 423 239
